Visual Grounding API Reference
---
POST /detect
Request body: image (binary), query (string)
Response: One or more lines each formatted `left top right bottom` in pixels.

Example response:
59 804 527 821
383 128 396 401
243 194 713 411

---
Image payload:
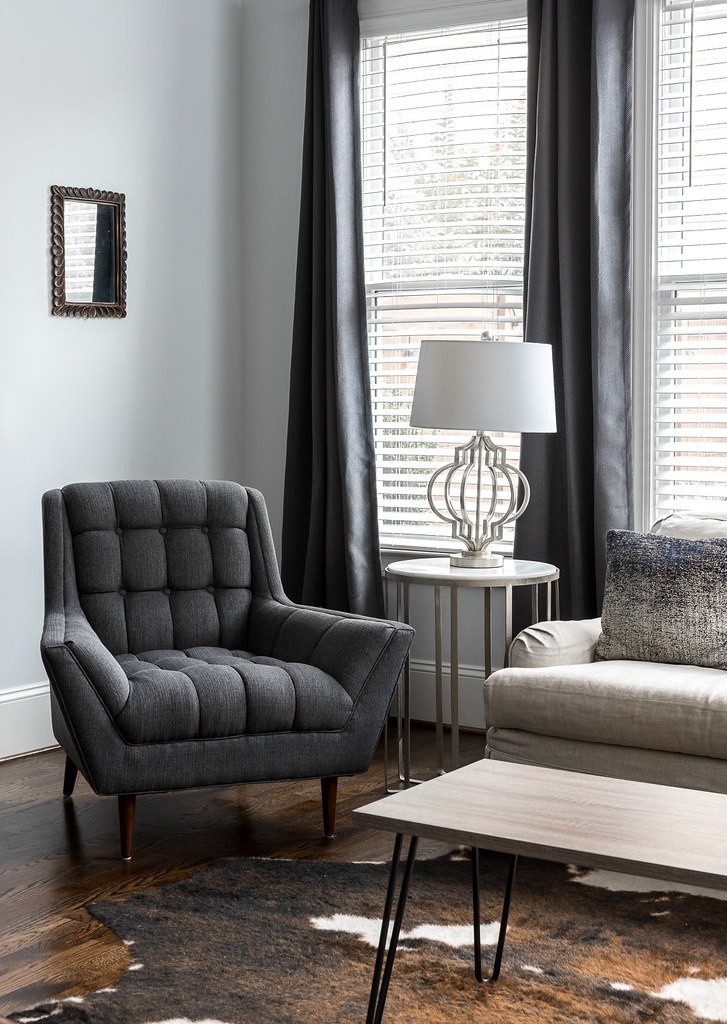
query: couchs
40 478 416 860
483 511 727 794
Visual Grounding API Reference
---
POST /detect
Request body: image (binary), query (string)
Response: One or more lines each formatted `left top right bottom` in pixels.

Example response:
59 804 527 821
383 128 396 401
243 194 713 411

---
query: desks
351 758 727 1024
384 558 560 794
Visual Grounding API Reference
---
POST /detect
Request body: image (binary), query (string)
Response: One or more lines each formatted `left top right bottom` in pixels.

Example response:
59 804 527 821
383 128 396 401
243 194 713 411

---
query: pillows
593 528 727 672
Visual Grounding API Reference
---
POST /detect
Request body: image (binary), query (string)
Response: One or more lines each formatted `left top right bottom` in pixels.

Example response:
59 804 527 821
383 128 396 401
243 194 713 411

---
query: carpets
4 844 727 1024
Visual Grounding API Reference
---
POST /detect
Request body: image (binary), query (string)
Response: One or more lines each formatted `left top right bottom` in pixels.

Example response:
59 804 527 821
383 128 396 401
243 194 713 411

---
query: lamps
409 331 557 569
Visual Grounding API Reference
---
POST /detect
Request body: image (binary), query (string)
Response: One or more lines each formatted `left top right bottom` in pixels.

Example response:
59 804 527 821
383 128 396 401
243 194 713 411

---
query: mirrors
50 185 127 318
